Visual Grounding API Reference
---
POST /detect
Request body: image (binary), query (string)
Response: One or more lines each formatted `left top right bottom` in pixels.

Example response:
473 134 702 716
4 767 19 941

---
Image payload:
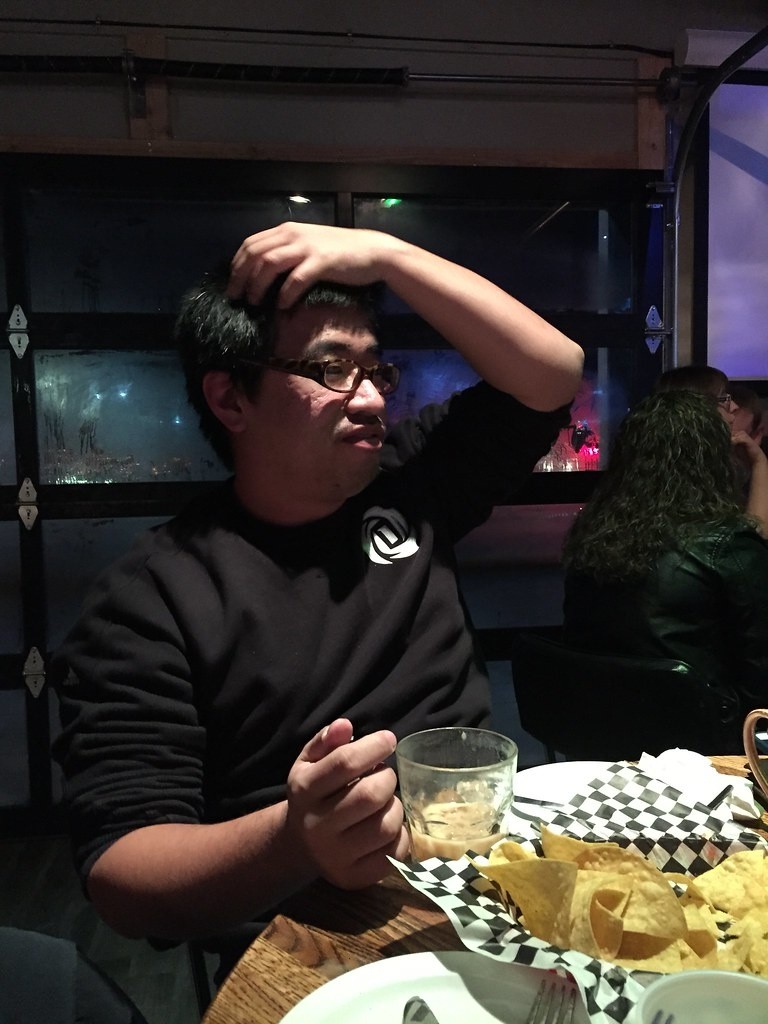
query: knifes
402 996 440 1024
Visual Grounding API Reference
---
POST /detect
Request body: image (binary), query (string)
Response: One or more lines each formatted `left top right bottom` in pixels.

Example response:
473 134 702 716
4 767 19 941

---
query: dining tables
197 755 768 1023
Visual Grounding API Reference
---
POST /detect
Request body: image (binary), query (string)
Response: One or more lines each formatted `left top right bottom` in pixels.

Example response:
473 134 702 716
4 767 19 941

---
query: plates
278 951 591 1024
506 762 733 840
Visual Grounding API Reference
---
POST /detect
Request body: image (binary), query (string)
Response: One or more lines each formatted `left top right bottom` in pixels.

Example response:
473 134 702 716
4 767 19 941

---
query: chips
467 825 768 981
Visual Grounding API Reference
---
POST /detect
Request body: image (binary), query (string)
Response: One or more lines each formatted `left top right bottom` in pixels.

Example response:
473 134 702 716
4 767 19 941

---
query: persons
562 390 768 754
731 387 768 459
45 223 585 980
651 365 768 540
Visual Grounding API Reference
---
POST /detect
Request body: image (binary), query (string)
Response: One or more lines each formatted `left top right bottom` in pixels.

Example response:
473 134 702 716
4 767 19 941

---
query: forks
526 979 576 1024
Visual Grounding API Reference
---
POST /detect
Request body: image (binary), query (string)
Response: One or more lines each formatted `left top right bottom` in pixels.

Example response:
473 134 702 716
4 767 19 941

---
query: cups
637 970 768 1024
397 727 518 864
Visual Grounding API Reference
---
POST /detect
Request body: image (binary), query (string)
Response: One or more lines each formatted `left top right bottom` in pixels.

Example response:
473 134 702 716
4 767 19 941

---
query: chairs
509 638 569 763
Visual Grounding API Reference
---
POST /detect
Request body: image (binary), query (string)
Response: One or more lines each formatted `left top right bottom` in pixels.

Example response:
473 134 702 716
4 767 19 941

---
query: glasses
238 348 402 396
715 393 731 403
